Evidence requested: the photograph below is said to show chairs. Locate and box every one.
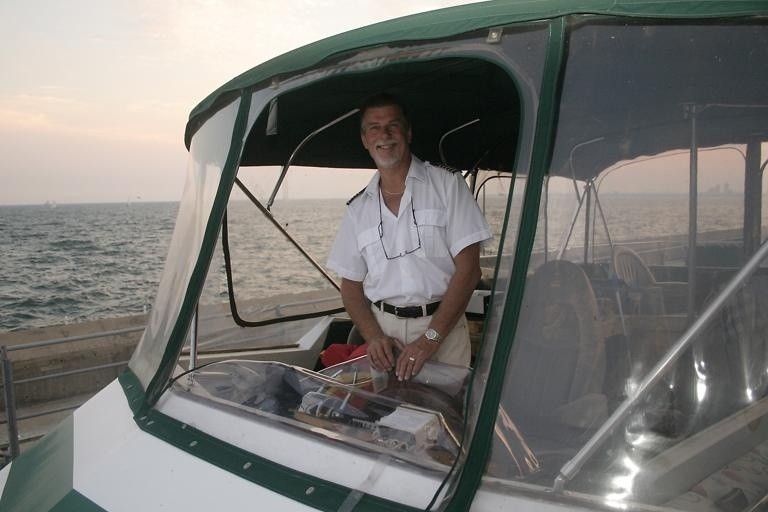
[492,258,608,480]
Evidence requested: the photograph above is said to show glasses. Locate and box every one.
[377,221,422,260]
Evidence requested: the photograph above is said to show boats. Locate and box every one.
[0,1,767,510]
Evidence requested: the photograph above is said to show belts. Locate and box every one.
[372,299,442,318]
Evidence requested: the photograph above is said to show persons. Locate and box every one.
[325,89,494,399]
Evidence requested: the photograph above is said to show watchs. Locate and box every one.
[424,327,443,344]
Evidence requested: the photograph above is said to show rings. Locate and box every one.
[409,357,415,362]
